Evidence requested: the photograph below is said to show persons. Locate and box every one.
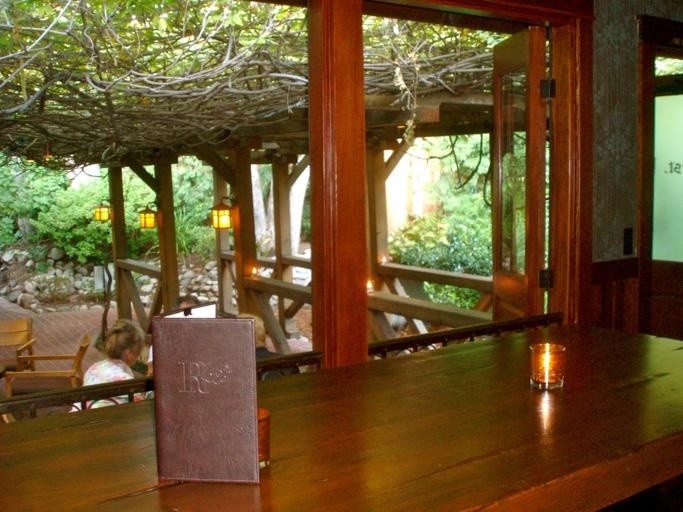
[66,318,145,416]
[236,313,301,380]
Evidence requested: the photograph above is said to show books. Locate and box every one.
[150,302,260,483]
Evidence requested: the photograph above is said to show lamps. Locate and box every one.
[90,196,160,229]
[208,192,238,231]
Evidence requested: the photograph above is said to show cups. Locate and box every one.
[530,342,566,390]
[257,407,271,469]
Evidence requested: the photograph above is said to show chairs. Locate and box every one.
[0,316,91,398]
